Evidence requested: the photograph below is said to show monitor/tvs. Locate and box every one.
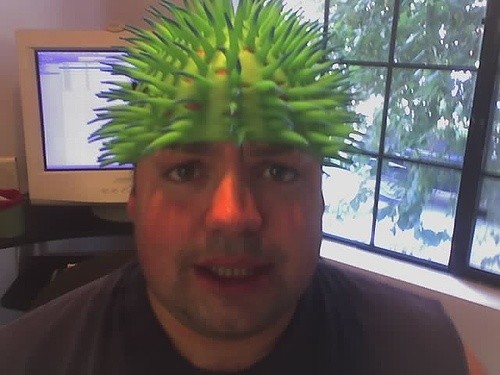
[15,28,153,223]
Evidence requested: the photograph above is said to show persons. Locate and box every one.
[0,0,469,375]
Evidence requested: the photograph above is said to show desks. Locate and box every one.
[0,205,139,314]
